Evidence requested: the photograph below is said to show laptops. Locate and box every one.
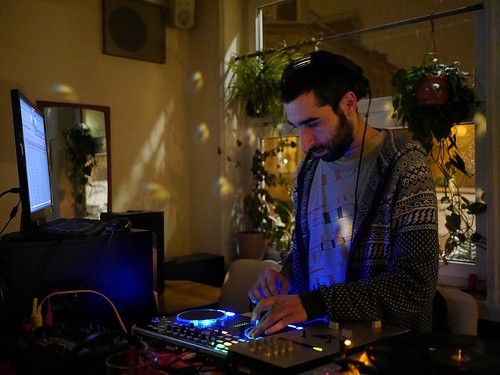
[10,88,107,237]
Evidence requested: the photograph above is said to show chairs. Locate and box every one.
[220,258,283,313]
[436,288,478,338]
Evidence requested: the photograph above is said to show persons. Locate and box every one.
[248,50,438,375]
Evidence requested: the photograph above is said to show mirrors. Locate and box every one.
[36,100,112,224]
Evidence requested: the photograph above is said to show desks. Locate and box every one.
[160,280,220,317]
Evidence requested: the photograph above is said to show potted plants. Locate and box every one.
[224,41,305,153]
[60,122,104,218]
[218,133,298,262]
[391,52,487,264]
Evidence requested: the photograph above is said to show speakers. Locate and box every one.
[169,0,195,29]
[100,210,166,297]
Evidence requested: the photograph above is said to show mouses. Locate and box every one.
[106,217,132,233]
